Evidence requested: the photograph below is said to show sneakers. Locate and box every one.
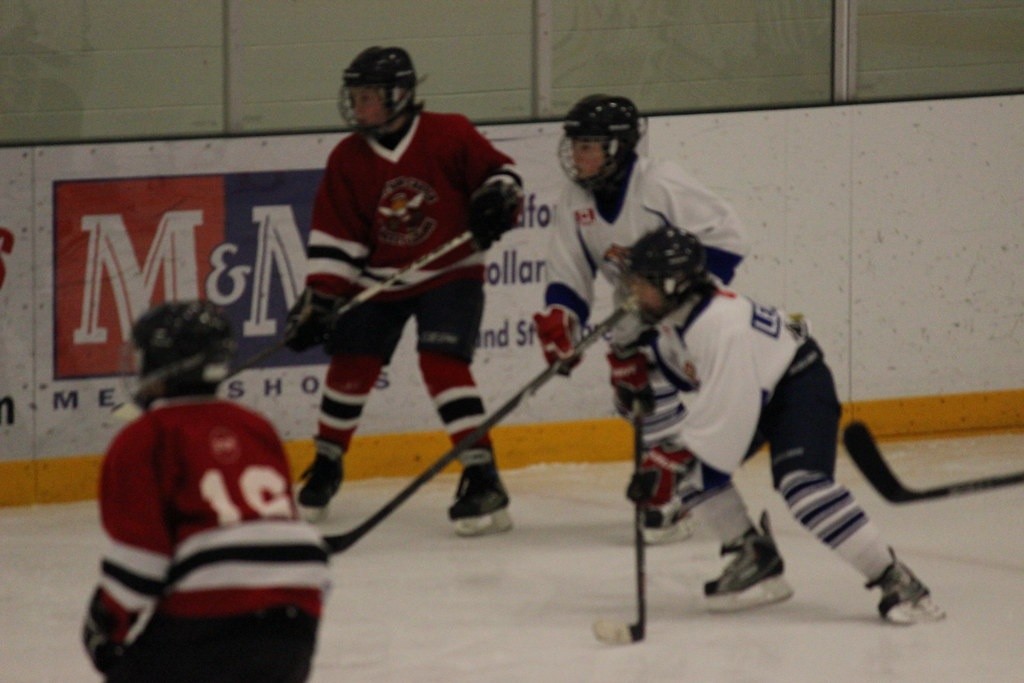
[866,545,944,623]
[635,494,692,543]
[294,435,345,521]
[447,447,510,535]
[702,510,794,613]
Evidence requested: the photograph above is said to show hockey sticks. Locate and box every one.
[843,421,1024,502]
[220,227,474,388]
[593,398,647,643]
[319,308,625,555]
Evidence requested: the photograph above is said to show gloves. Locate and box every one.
[534,305,582,374]
[282,286,344,349]
[628,440,696,504]
[467,176,523,251]
[608,349,655,413]
[83,616,121,671]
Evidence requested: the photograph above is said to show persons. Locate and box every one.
[282,45,526,536]
[84,299,333,683]
[533,94,750,543]
[626,224,947,627]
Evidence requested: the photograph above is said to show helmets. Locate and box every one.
[628,224,701,318]
[556,94,639,184]
[336,45,416,132]
[123,298,241,406]
